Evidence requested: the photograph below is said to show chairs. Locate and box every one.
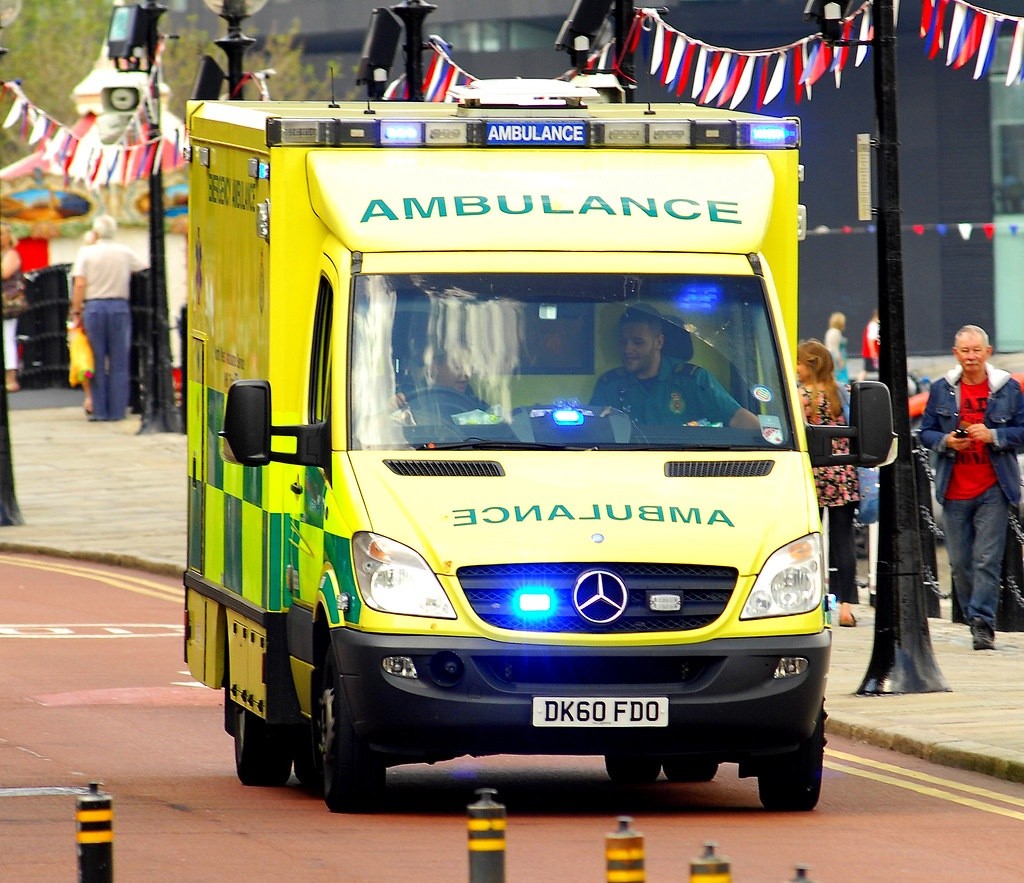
[660,313,692,359]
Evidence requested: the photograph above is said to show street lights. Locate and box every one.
[204,1,274,102]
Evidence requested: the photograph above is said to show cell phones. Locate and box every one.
[956,429,969,438]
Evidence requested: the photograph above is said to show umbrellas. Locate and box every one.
[0,112,187,216]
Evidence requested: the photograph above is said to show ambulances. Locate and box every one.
[179,74,899,809]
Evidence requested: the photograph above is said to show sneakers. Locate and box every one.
[969,616,997,650]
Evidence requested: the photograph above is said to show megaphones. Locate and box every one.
[102,72,149,114]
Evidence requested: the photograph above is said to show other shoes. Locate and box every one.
[838,612,859,627]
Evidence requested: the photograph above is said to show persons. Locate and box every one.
[0,223,23,392]
[68,215,152,422]
[366,336,519,441]
[586,303,760,429]
[858,309,879,382]
[825,313,850,383]
[797,340,861,627]
[918,324,1024,649]
